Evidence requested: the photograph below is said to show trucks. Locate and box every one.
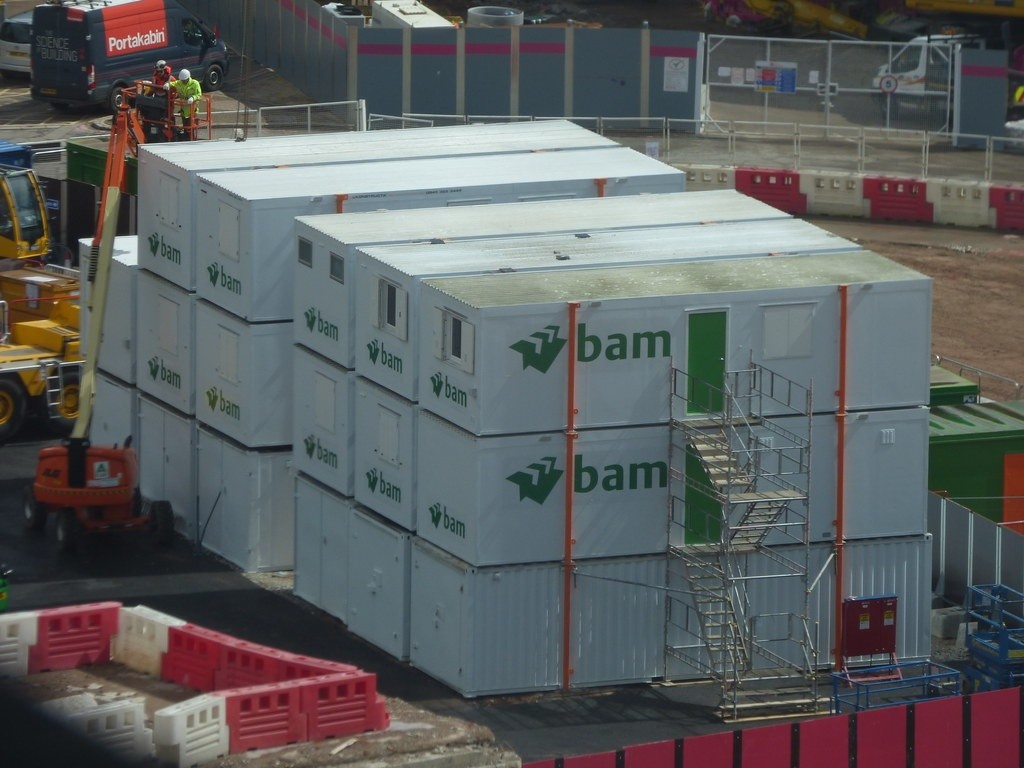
[876,35,988,105]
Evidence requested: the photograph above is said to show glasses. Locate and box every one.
[154,65,165,69]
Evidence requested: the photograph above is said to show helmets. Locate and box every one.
[156,60,166,71]
[178,69,190,81]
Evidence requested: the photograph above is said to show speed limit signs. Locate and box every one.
[881,75,897,93]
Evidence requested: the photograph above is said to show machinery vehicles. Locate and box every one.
[0,161,88,452]
[19,76,201,564]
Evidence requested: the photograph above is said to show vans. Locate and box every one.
[0,10,34,75]
[30,0,232,115]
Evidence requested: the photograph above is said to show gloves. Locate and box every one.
[163,83,170,91]
[187,97,193,105]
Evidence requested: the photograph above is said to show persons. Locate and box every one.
[163,69,202,134]
[146,59,176,125]
